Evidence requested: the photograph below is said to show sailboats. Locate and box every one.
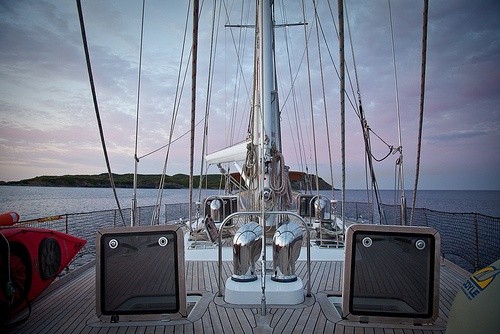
[0,0,499,334]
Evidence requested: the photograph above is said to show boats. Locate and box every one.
[0,226,88,322]
